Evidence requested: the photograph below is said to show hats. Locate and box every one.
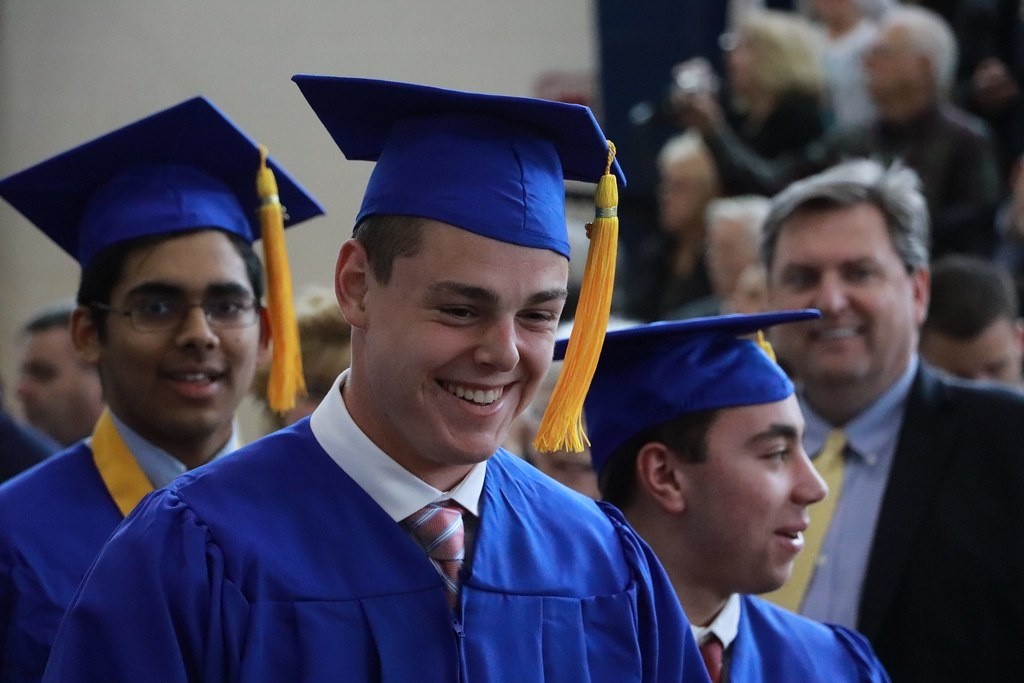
[1,95,327,416]
[554,308,822,475]
[291,74,628,453]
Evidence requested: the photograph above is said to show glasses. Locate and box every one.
[94,279,263,334]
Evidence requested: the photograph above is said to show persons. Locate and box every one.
[41,76,712,683]
[18,301,106,448]
[506,306,891,683]
[0,94,326,683]
[534,0,1024,682]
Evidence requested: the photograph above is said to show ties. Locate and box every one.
[698,636,723,683]
[758,428,846,614]
[404,503,471,615]
